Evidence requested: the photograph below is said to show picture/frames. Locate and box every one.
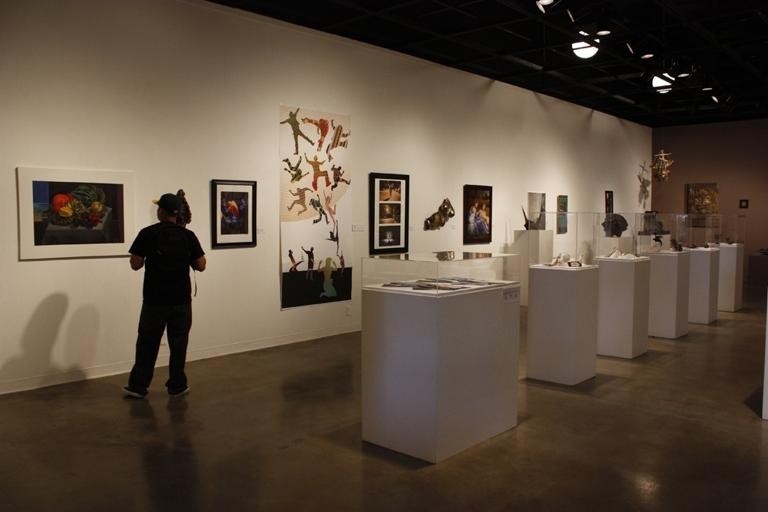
[16,164,137,261]
[367,172,410,255]
[211,178,257,250]
[462,183,494,246]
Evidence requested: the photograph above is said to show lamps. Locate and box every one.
[535,0,734,105]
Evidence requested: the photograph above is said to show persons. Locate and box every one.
[121,189,206,398]
[468,199,490,237]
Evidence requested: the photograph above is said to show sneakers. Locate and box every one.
[168,385,191,398]
[122,386,145,399]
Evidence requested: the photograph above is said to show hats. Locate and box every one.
[153,193,179,206]
